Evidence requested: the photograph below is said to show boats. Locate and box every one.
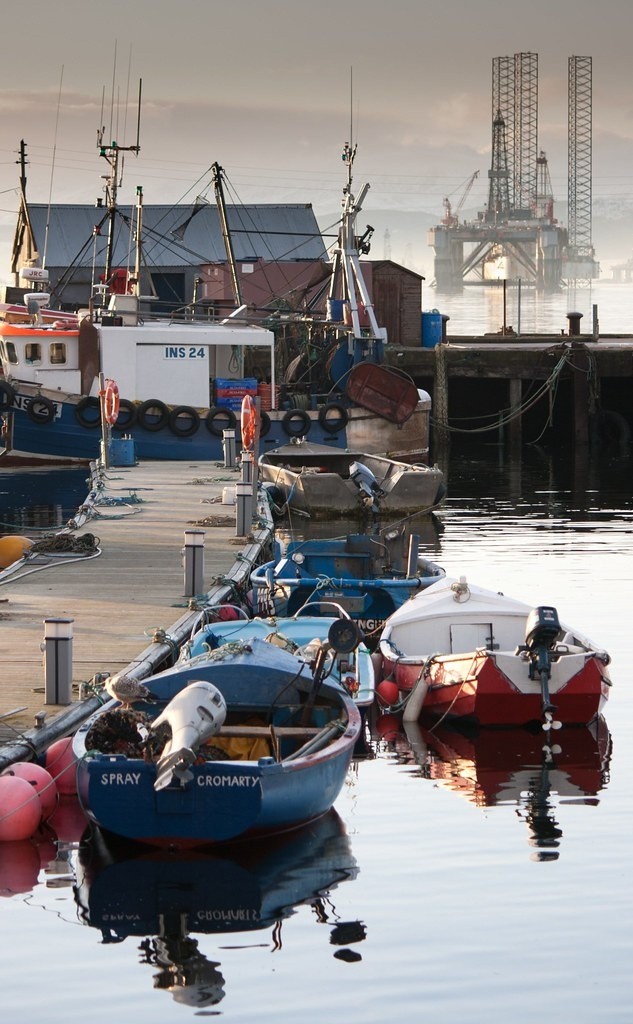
[1,34,433,467]
[369,574,615,732]
[70,633,364,857]
[175,602,375,715]
[245,519,448,654]
[257,433,448,522]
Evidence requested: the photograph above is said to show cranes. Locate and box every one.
[441,169,482,228]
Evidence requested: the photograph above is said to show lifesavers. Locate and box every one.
[138,399,170,432]
[76,396,102,428]
[113,399,138,430]
[0,380,15,410]
[206,407,237,436]
[283,408,312,438]
[27,396,54,424]
[259,409,271,437]
[169,406,200,437]
[267,486,283,518]
[53,319,88,328]
[319,402,349,434]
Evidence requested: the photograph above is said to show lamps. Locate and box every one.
[184,529,206,546]
[44,618,74,639]
[241,450,254,461]
[236,480,252,497]
[223,429,235,439]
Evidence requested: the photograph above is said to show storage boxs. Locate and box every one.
[326,299,375,326]
[213,378,321,414]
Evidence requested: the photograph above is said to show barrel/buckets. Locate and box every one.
[107,438,135,467]
[327,299,375,327]
[422,313,442,347]
